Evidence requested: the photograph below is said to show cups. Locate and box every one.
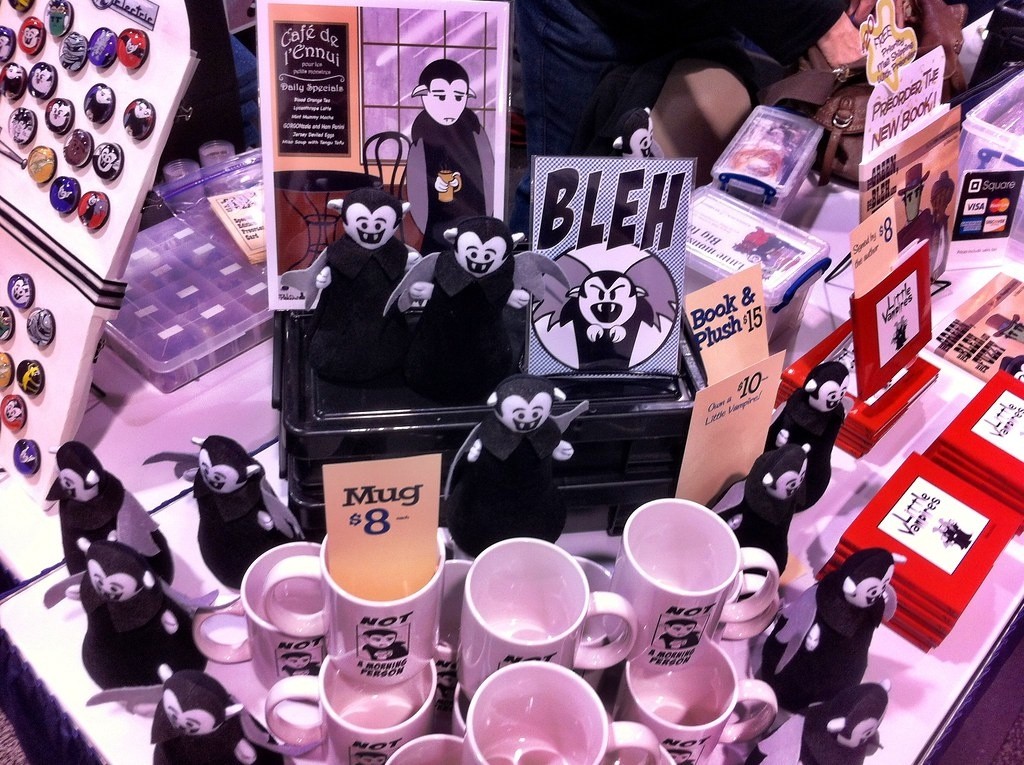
[274,169,383,276]
[194,498,780,765]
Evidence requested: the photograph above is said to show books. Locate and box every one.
[858,103,1024,282]
[923,272,1024,392]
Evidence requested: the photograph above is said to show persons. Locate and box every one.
[509,0,914,253]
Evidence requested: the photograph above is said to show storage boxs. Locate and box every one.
[682,184,832,345]
[100,215,275,393]
[968,0,1024,88]
[957,69,1024,285]
[710,104,824,219]
